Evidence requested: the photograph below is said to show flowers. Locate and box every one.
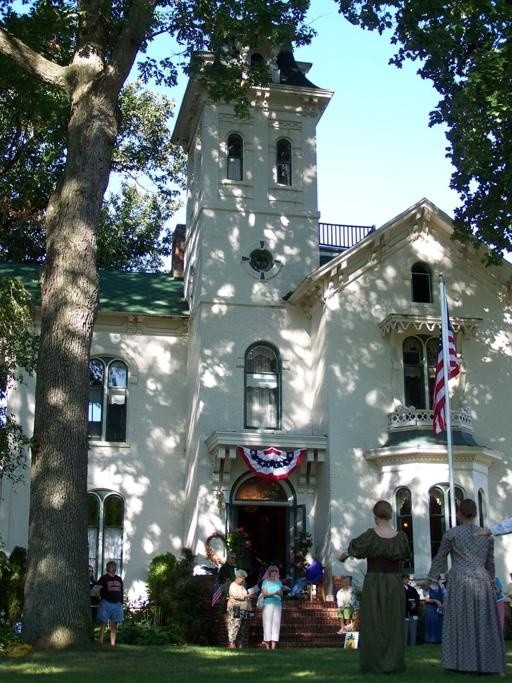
[287,531,313,578]
[226,526,252,570]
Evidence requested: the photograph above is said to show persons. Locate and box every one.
[94,561,124,647]
[422,496,508,675]
[491,575,506,634]
[284,552,322,600]
[87,564,100,625]
[472,514,512,539]
[224,567,256,648]
[335,575,357,634]
[421,574,445,643]
[260,562,288,649]
[400,573,421,647]
[216,552,237,618]
[333,499,416,674]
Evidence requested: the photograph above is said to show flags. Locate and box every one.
[432,292,461,436]
[211,585,222,607]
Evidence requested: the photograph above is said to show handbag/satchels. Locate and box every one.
[232,604,241,619]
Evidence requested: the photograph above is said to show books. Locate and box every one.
[247,583,260,594]
[279,584,291,590]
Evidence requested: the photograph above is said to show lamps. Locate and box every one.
[403,518,409,527]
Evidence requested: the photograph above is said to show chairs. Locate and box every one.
[308,567,327,602]
